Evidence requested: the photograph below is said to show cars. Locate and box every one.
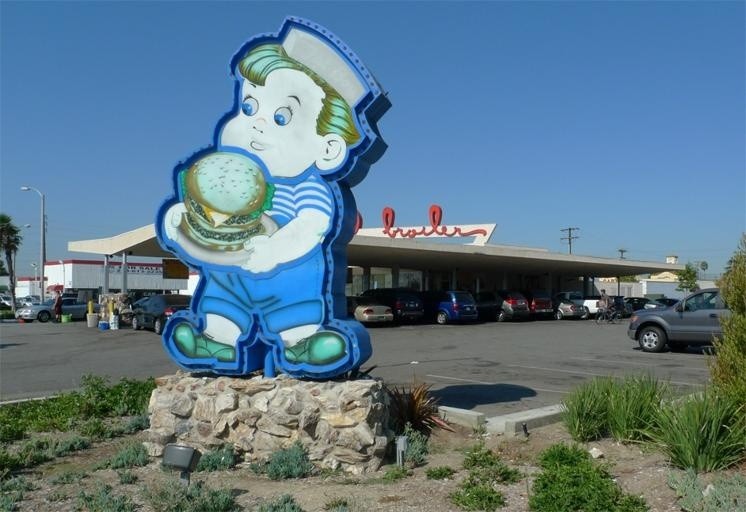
[0,292,155,323]
[624,287,731,354]
[128,293,194,335]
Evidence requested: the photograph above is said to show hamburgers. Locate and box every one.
[181,150,274,251]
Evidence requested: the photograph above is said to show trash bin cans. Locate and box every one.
[87,314,99,327]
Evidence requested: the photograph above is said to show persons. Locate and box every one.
[153,16,393,379]
[52,291,63,322]
[599,288,615,322]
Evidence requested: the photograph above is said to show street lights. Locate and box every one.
[56,259,65,293]
[29,261,39,295]
[12,220,31,285]
[19,185,46,303]
